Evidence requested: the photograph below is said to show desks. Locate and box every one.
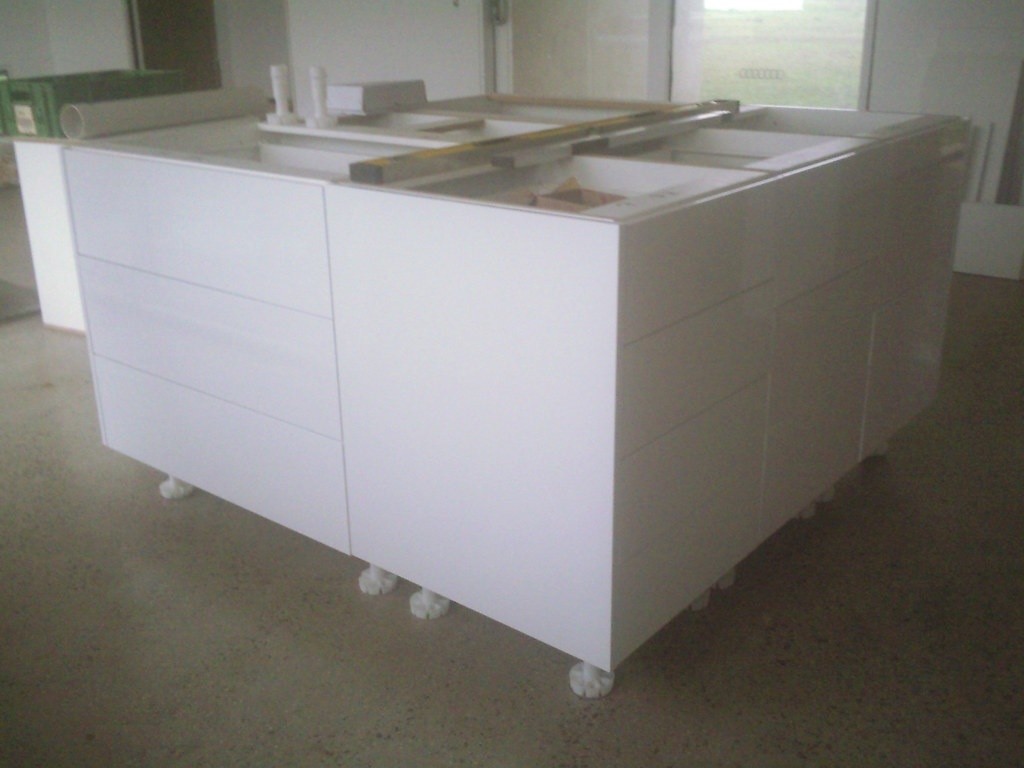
[60,110,979,675]
